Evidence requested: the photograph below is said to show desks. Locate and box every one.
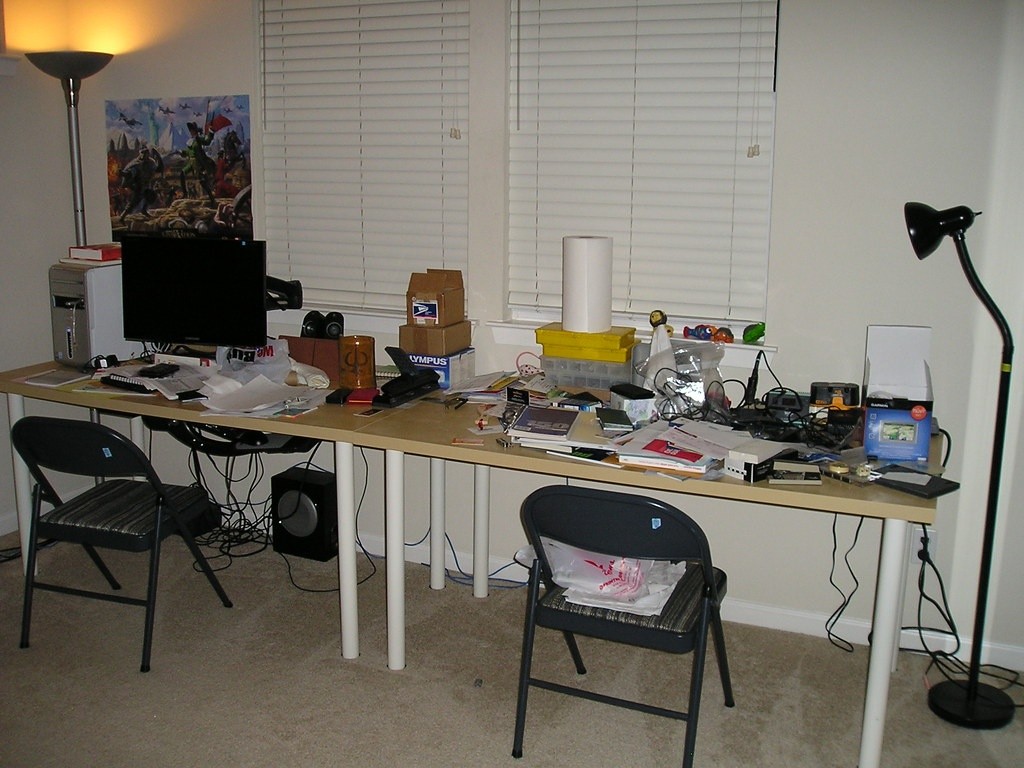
[1,346,949,768]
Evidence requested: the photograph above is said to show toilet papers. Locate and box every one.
[562,234,614,334]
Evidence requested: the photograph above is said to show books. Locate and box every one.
[69,243,122,262]
[92,360,208,403]
[444,371,744,483]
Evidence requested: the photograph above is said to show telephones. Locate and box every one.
[372,368,442,408]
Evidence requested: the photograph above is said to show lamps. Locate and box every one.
[905,200,1017,730]
[23,50,114,266]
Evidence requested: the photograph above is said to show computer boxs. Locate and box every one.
[48,263,155,366]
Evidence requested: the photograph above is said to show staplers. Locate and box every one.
[767,459,823,486]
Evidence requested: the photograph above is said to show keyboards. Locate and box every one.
[187,422,246,443]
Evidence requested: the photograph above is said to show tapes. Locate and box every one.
[829,460,850,473]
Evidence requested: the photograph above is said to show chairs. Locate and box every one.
[512,484,735,767]
[8,416,234,672]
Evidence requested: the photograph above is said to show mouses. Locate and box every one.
[247,432,268,446]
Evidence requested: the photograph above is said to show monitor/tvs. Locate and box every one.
[118,231,302,349]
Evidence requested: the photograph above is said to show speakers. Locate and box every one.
[270,467,339,561]
[299,310,344,339]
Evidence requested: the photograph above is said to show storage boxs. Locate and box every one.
[398,268,476,388]
[862,325,934,461]
[536,322,642,392]
